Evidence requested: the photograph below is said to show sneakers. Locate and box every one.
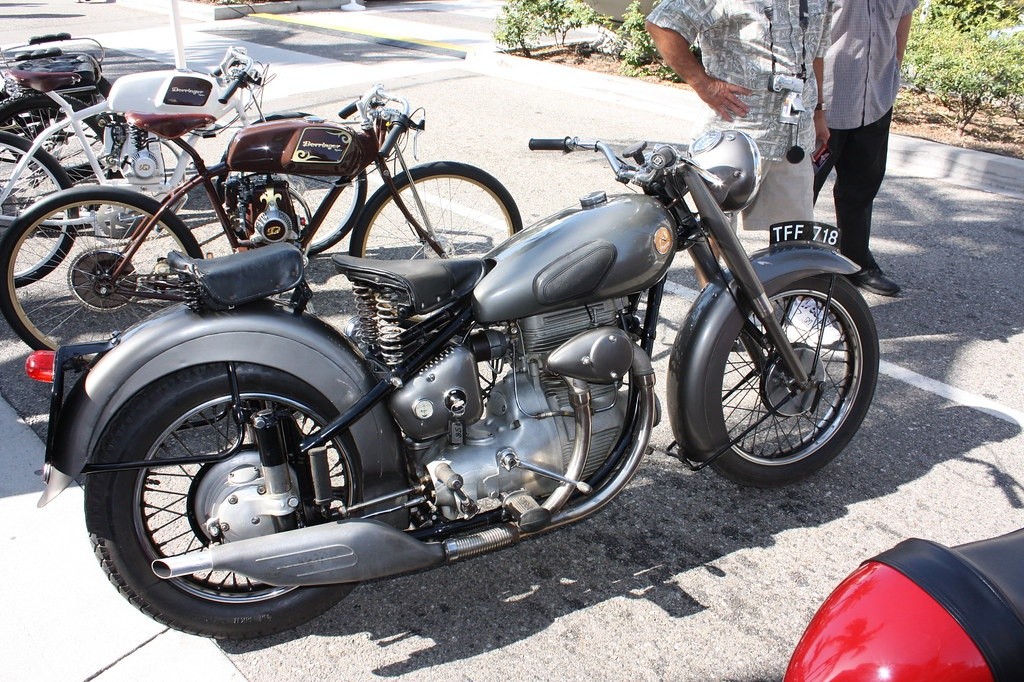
[789,298,840,348]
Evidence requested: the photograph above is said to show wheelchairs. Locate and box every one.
[0,32,128,230]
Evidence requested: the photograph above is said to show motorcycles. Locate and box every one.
[25,129,881,639]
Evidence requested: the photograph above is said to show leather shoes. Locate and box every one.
[849,263,901,296]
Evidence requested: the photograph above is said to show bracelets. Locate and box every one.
[815,103,826,111]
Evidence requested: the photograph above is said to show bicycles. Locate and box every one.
[0,46,368,288]
[1,82,522,366]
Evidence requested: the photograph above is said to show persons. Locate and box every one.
[641,0,844,353]
[809,0,923,294]
